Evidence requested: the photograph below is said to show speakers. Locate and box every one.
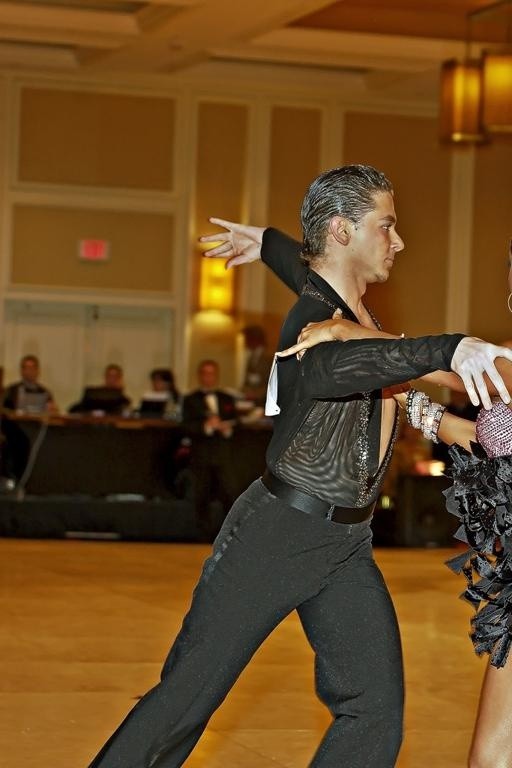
[395,475,461,545]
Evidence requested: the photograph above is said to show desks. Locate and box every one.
[1,409,462,554]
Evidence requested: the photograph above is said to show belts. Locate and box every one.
[259,466,380,528]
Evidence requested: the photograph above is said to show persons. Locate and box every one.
[431,392,488,460]
[86,165,512,768]
[1,325,277,426]
[270,242,512,768]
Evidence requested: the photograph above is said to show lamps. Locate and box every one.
[441,0,486,146]
[476,0,512,134]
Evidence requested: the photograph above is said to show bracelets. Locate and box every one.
[406,383,448,446]
